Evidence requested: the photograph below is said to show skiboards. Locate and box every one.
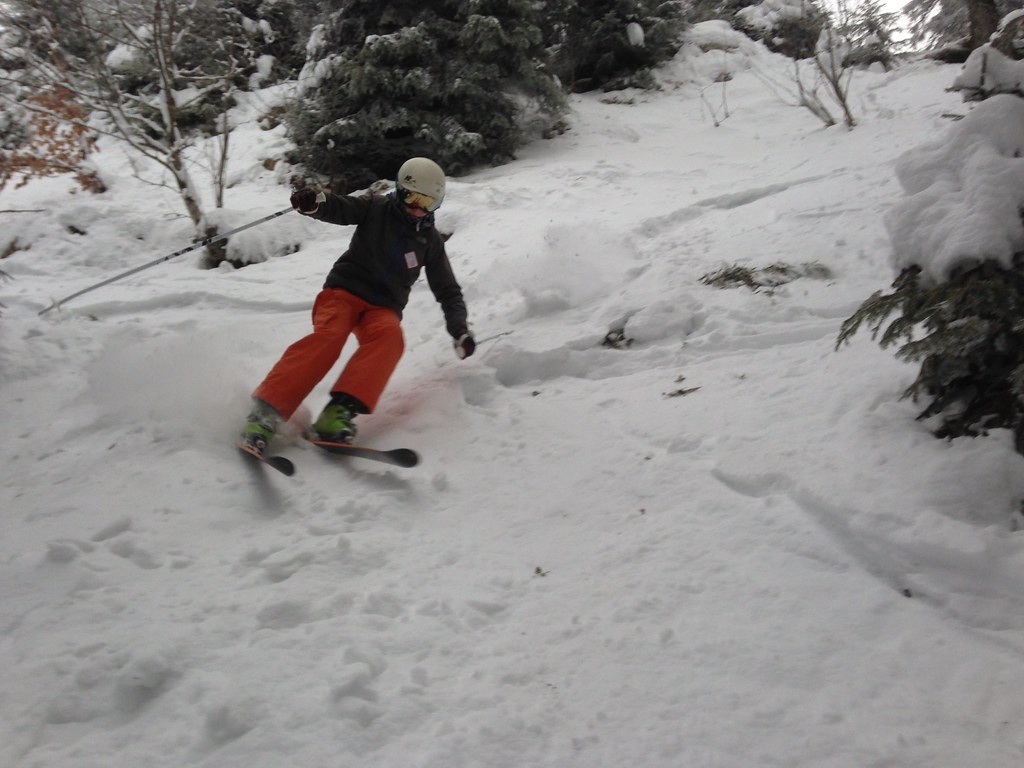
[234,435,422,477]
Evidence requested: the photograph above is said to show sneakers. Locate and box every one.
[311,397,363,441]
[241,402,278,444]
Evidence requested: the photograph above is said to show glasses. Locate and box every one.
[402,191,441,213]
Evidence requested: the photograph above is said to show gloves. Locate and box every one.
[291,188,318,213]
[448,320,476,361]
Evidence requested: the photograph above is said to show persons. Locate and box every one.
[239,157,476,456]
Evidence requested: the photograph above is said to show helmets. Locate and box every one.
[398,157,446,203]
